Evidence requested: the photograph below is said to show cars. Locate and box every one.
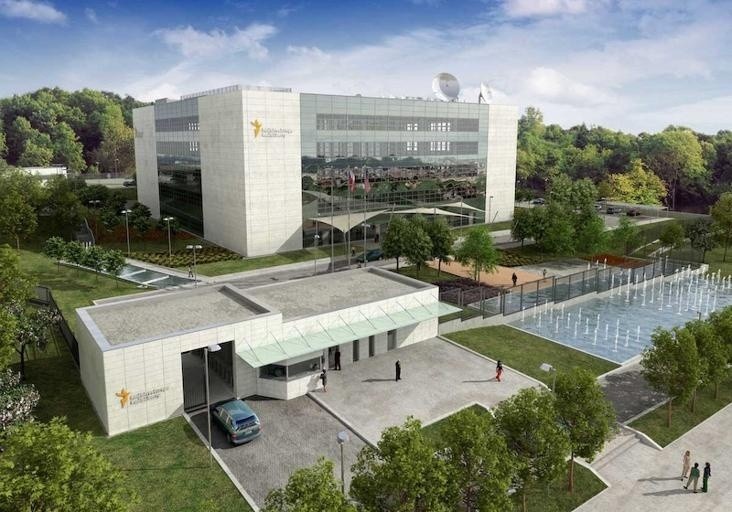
[357,249,385,263]
[303,165,474,189]
[594,204,602,210]
[213,400,263,444]
[534,198,546,204]
[607,207,623,213]
[627,209,640,216]
[123,181,136,187]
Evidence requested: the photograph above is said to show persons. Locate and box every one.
[680,450,691,481]
[543,268,547,277]
[320,368,329,392]
[333,348,341,370]
[683,462,700,493]
[699,462,711,492]
[511,272,518,286]
[395,359,404,382]
[494,359,505,382]
[312,363,319,371]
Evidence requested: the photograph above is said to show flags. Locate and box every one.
[364,167,371,194]
[348,166,357,194]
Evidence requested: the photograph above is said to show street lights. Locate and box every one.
[362,223,371,267]
[121,209,133,256]
[337,432,347,504]
[90,200,100,245]
[314,235,320,275]
[163,217,174,263]
[186,245,202,286]
[204,345,222,468]
[540,363,556,393]
